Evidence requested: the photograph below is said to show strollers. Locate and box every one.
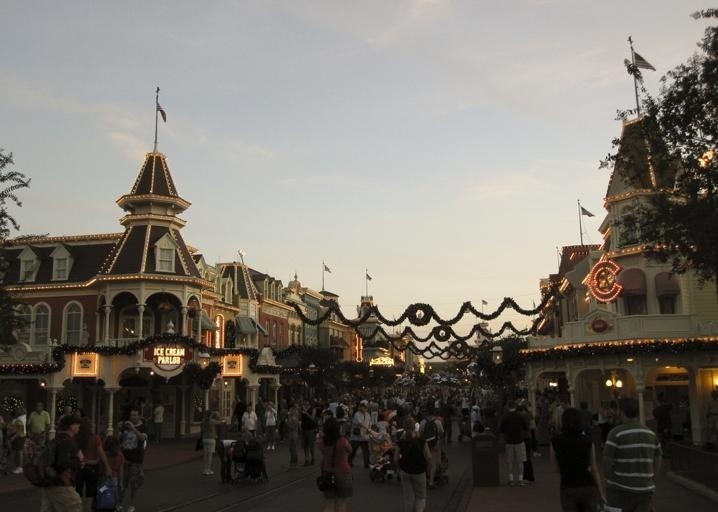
[366,430,399,484]
[229,436,268,487]
[455,413,471,442]
[427,449,450,488]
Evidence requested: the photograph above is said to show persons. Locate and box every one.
[8,400,164,512]
[548,396,672,512]
[200,386,537,510]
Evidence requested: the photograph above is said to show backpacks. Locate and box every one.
[24,438,74,488]
[422,418,439,446]
[93,475,118,512]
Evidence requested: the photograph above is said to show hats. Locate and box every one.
[59,414,83,429]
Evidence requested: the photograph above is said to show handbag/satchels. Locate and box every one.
[315,474,337,492]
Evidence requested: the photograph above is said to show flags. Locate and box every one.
[483,300,488,305]
[324,265,331,273]
[633,51,657,72]
[581,206,595,218]
[367,274,372,280]
[157,96,166,123]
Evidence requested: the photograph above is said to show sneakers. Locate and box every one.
[506,479,536,487]
[201,468,214,476]
[12,466,24,474]
[127,505,137,512]
